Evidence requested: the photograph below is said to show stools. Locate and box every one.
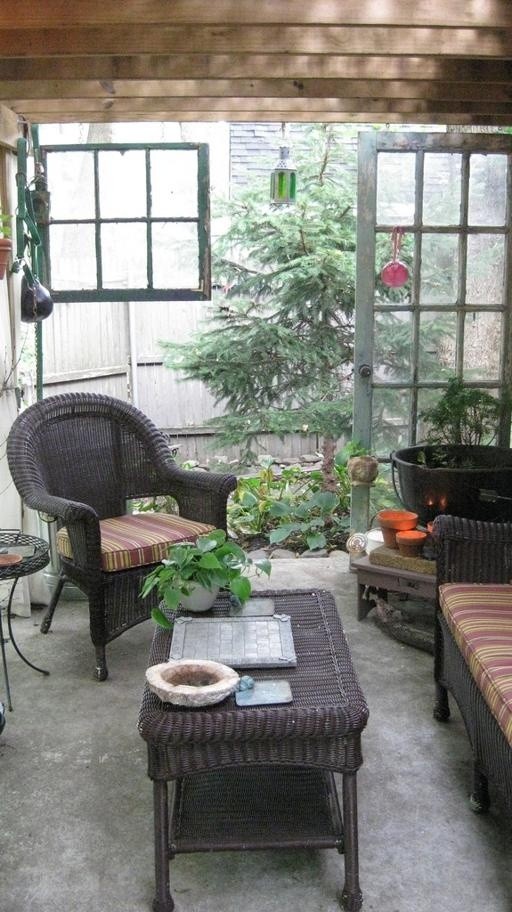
[351,555,436,622]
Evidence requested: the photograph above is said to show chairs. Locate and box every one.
[7,390,237,682]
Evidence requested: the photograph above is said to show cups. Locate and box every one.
[393,529,427,553]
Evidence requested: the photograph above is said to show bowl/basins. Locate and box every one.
[144,659,242,709]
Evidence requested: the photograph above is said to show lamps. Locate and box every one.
[268,146,297,206]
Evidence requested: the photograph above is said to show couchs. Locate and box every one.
[429,515,512,814]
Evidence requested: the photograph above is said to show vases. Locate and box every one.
[395,531,427,557]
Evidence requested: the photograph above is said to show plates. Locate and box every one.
[0,553,23,567]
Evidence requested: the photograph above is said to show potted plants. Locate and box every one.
[390,375,512,531]
[136,528,272,628]
[0,207,14,281]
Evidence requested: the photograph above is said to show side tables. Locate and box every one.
[0,531,55,715]
